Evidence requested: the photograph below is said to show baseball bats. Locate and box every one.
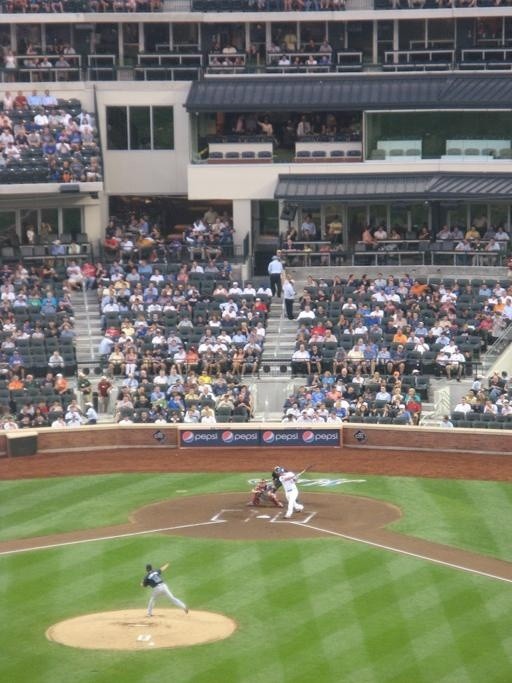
[296,465,312,476]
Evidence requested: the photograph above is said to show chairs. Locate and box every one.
[1,96,101,184]
[207,121,361,161]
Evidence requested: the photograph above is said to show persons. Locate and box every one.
[248,0,345,11]
[140,562,188,617]
[282,215,512,427]
[233,113,362,144]
[2,41,76,82]
[205,23,333,66]
[274,466,304,519]
[1,206,272,431]
[268,250,285,297]
[0,87,102,182]
[3,1,161,13]
[245,479,283,507]
[392,1,502,8]
[271,464,298,503]
